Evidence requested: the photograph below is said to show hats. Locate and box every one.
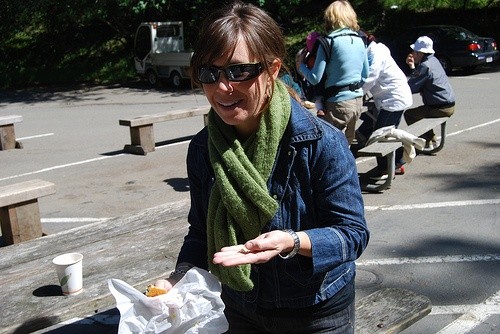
[410,36,435,53]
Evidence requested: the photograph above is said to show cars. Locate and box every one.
[382,23,500,75]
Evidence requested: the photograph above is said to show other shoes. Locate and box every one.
[368,168,388,180]
[395,164,404,174]
[424,140,433,151]
[317,110,325,115]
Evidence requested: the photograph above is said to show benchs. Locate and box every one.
[0,115,24,151]
[354,117,450,189]
[0,178,58,244]
[355,287,434,334]
[119,106,213,155]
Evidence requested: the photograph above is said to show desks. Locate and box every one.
[0,199,196,334]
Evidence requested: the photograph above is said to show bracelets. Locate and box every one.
[278,229,300,260]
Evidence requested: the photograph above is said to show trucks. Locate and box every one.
[131,19,195,90]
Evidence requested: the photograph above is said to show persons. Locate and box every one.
[280,0,455,175]
[153,4,370,334]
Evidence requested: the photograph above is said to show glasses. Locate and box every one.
[195,61,272,85]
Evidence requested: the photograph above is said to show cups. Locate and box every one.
[52,252,83,296]
